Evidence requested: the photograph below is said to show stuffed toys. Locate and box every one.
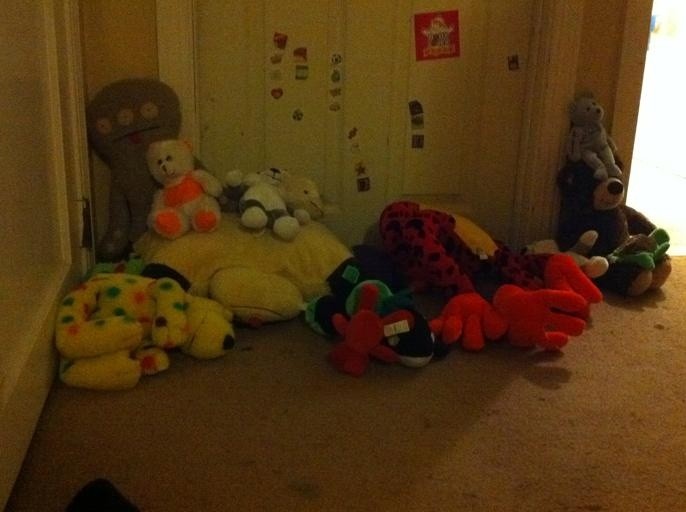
[51,77,671,391]
